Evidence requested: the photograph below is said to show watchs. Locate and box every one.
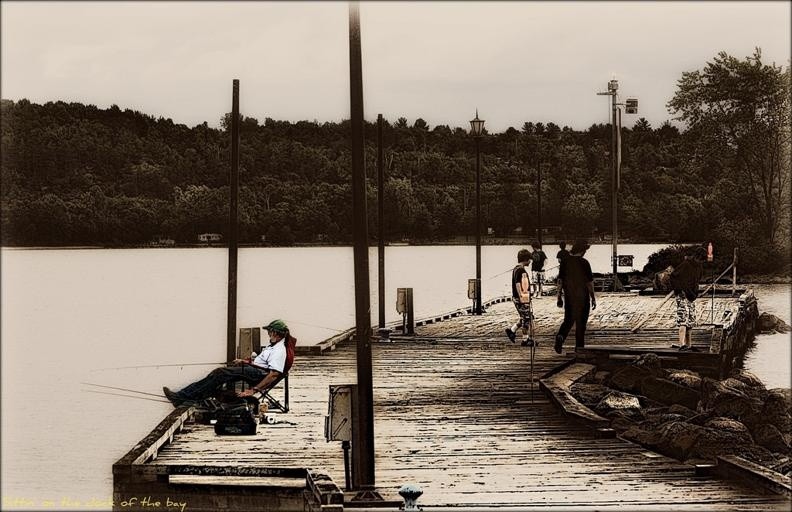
[253,385,259,392]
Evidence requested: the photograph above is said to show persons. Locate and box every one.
[668,246,707,352]
[556,242,568,264]
[555,240,596,353]
[505,249,538,347]
[529,241,548,298]
[162,318,290,408]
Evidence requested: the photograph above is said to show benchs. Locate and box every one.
[544,277,613,292]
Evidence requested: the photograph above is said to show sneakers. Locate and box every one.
[556,335,563,354]
[163,386,184,408]
[505,328,516,344]
[521,338,538,346]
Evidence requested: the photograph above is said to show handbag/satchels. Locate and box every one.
[214,406,257,435]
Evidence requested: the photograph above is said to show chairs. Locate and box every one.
[227,330,296,413]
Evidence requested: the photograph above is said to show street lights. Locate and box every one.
[470,107,486,317]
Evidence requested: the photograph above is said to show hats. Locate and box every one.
[570,243,590,254]
[262,319,288,331]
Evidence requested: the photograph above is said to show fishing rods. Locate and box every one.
[489,264,513,279]
[85,359,253,370]
[277,319,356,336]
[79,380,224,413]
